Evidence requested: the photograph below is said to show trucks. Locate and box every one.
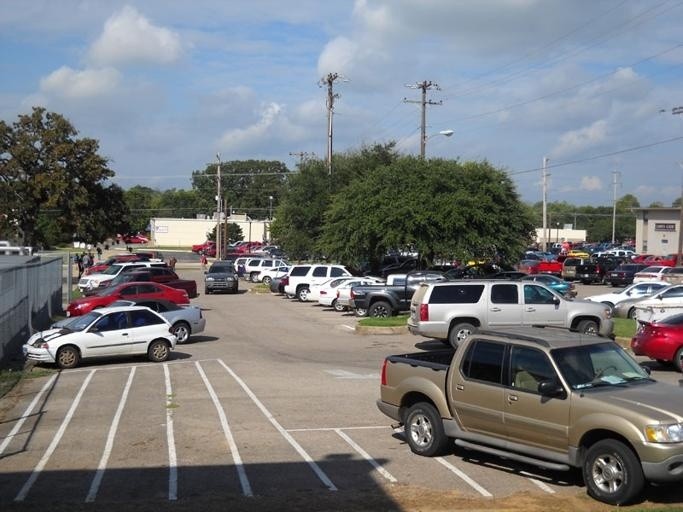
[379,332,683,503]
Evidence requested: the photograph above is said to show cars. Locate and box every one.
[0,229,209,366]
[584,265,683,374]
[443,241,682,299]
[192,237,451,316]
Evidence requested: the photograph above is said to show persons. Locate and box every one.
[72,248,95,280]
[598,306,616,339]
[166,255,177,272]
[198,251,209,272]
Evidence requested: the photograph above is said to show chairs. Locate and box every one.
[515,358,546,392]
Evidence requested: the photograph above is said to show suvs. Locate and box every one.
[410,281,615,348]
